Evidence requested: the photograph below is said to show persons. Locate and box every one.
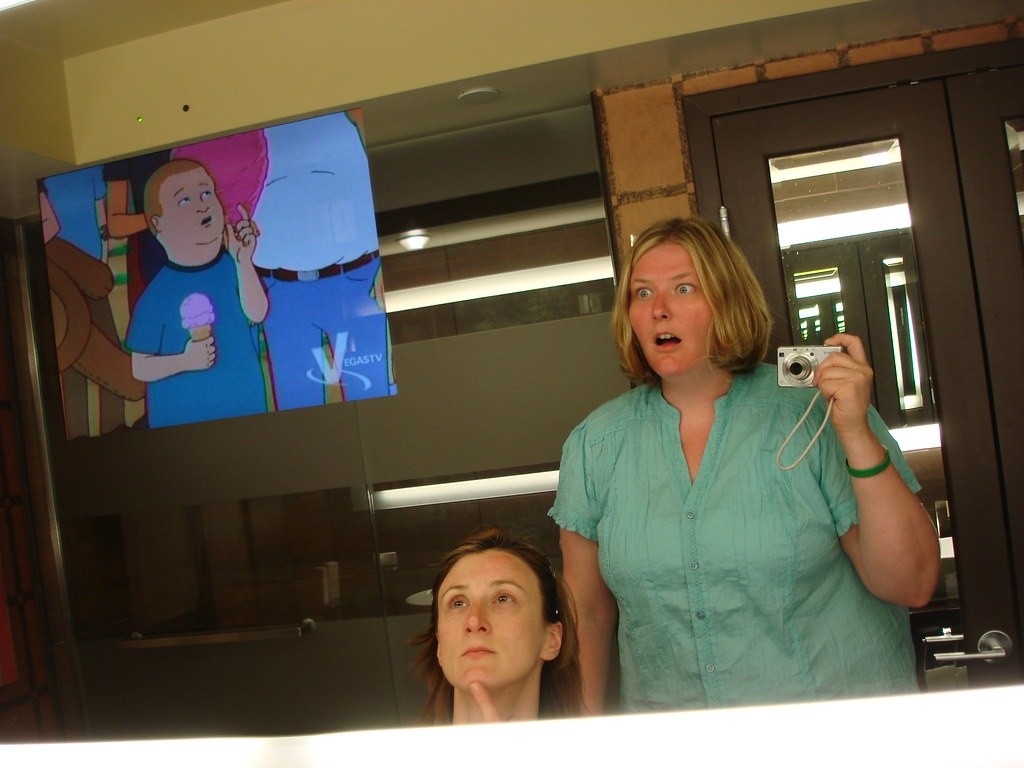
[418,527,585,726]
[548,217,939,718]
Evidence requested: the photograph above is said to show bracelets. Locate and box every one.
[846,445,890,477]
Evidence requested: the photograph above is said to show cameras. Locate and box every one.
[777,344,846,388]
